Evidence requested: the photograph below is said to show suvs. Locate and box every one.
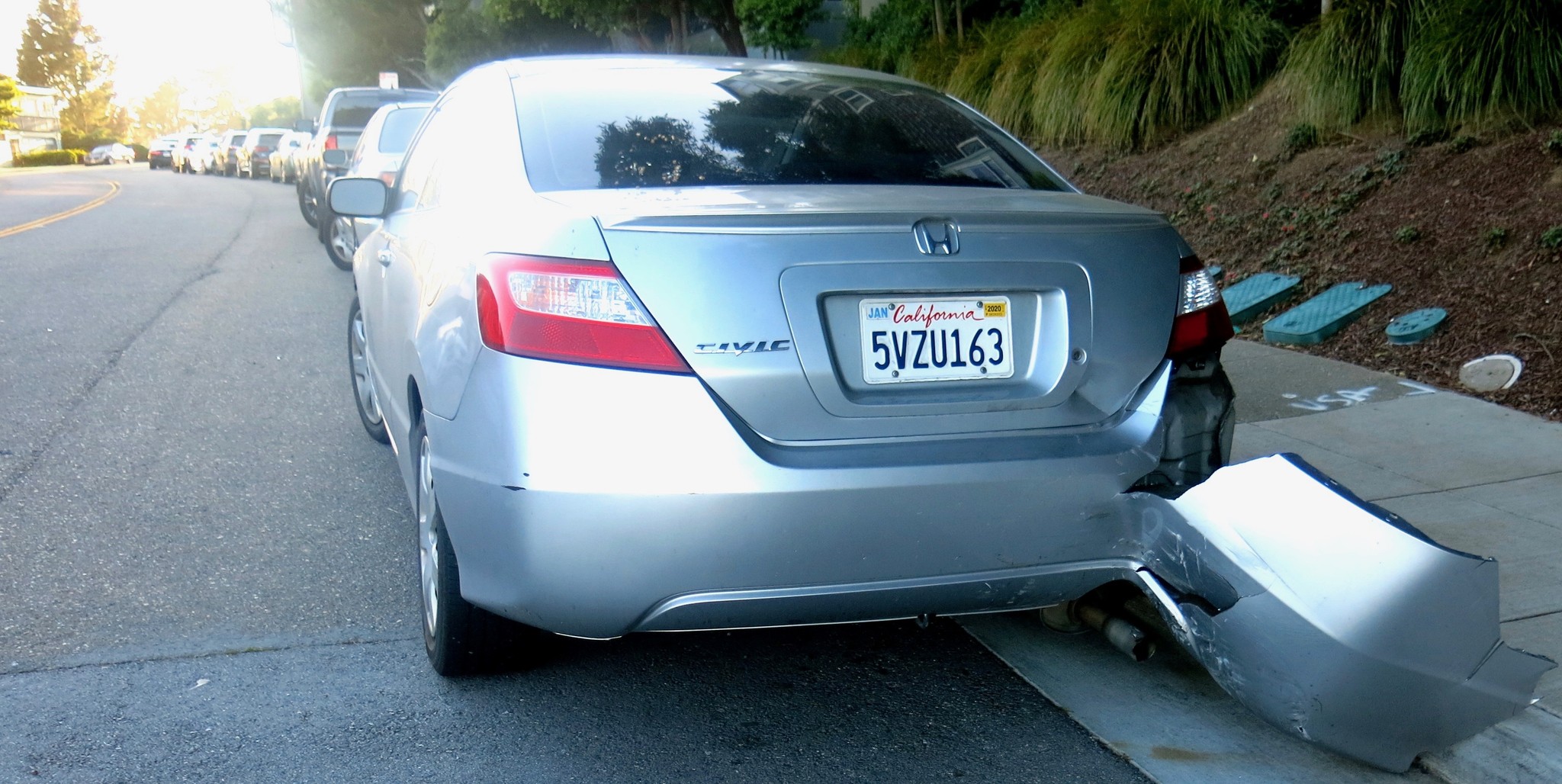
[293,83,445,245]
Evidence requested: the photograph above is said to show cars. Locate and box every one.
[317,96,441,272]
[144,120,314,185]
[321,48,1558,777]
[83,142,136,166]
